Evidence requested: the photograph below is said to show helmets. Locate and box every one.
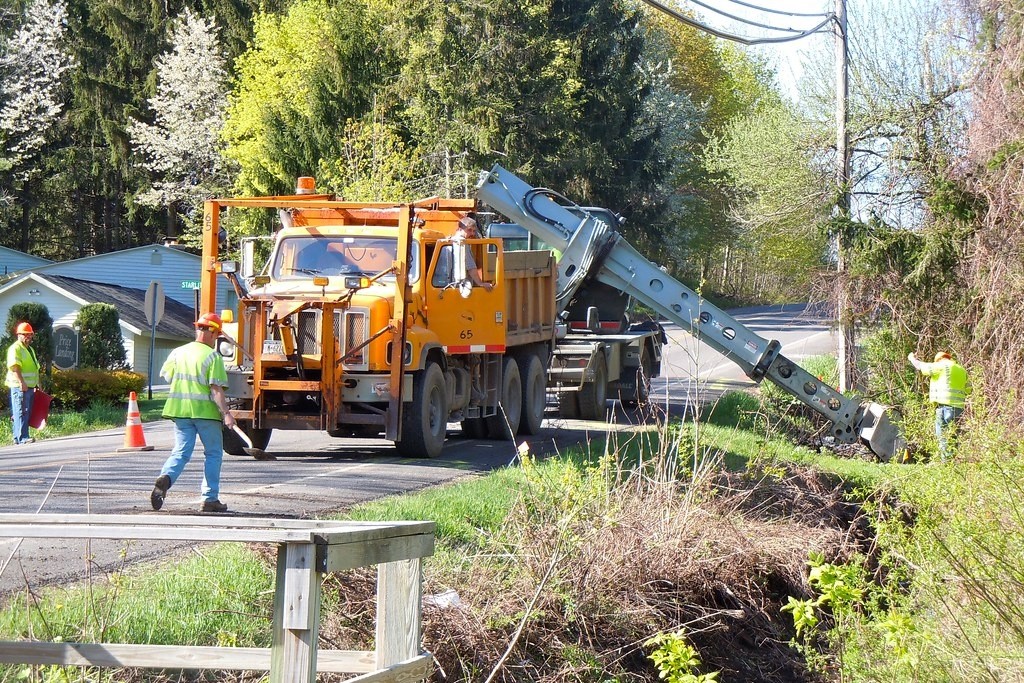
[193,312,224,336]
[934,351,953,362]
[16,323,34,334]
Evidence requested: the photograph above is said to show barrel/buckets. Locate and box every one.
[11,388,52,430]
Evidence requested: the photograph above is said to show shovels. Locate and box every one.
[233,425,277,461]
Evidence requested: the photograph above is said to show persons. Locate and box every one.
[432,217,495,293]
[908,352,973,463]
[5,323,42,444]
[150,312,237,512]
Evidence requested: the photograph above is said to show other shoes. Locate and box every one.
[13,437,36,445]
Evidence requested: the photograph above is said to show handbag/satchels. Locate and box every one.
[10,387,53,429]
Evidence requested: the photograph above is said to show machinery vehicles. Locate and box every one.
[487,207,909,462]
[200,177,557,459]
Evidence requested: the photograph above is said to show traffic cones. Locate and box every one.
[117,391,154,452]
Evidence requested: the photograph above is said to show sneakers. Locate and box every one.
[200,500,228,512]
[151,475,171,510]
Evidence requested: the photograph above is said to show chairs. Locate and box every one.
[324,252,344,270]
